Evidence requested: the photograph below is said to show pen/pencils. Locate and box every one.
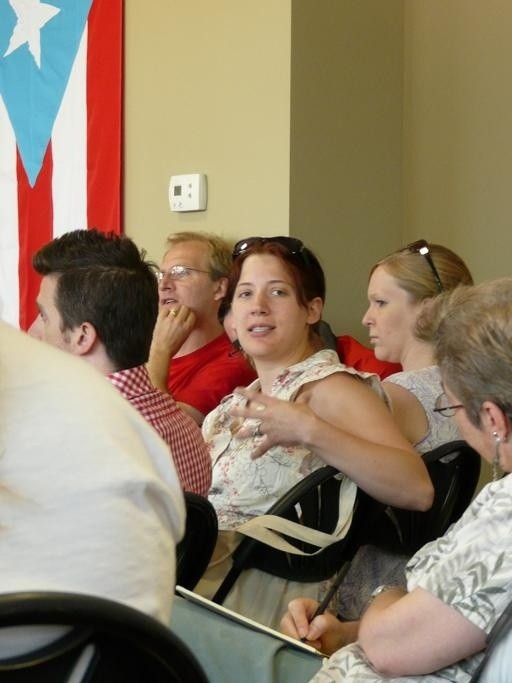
[300,560,351,643]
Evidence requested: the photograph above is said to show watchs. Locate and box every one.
[368,584,405,602]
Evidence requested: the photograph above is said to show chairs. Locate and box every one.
[0,590,209,682]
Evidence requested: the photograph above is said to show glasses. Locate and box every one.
[231,236,305,261]
[433,392,464,417]
[154,264,211,285]
[407,238,444,292]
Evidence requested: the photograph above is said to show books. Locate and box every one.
[175,582,331,661]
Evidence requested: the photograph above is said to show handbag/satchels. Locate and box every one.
[192,529,339,633]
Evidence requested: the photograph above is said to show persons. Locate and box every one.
[358,236,478,456]
[305,274,511,683]
[19,225,216,505]
[218,290,404,381]
[146,227,263,432]
[0,302,189,681]
[198,234,436,531]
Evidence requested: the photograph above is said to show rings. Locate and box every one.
[169,308,179,316]
[252,423,264,438]
